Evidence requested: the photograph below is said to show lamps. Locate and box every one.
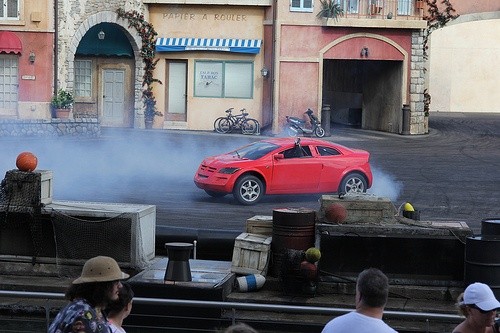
[261,66,271,79]
[98,24,105,39]
[29,50,36,63]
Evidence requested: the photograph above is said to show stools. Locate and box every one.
[164,242,194,282]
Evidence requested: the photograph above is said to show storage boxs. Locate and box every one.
[4,169,54,208]
[314,219,471,286]
[230,215,273,278]
[320,194,391,224]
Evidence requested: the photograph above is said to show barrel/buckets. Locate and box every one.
[272,208,317,276]
[463,218,500,303]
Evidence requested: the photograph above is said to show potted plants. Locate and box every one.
[317,0,345,23]
[50,89,75,118]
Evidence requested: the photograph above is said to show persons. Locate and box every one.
[449,284,500,333]
[48,255,132,333]
[218,323,258,333]
[321,268,399,333]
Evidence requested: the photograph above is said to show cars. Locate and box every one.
[193,135,373,206]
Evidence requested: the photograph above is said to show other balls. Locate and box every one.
[16,151,39,172]
[324,203,347,225]
[300,260,317,281]
[304,248,323,263]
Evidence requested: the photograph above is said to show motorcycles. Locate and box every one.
[282,108,325,137]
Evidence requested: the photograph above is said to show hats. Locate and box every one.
[72,255,130,284]
[458,282,500,311]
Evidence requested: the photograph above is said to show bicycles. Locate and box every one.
[214,107,260,135]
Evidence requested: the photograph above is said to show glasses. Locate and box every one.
[469,304,496,313]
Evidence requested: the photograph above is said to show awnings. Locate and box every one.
[155,36,262,55]
[0,31,23,56]
[74,23,133,58]
